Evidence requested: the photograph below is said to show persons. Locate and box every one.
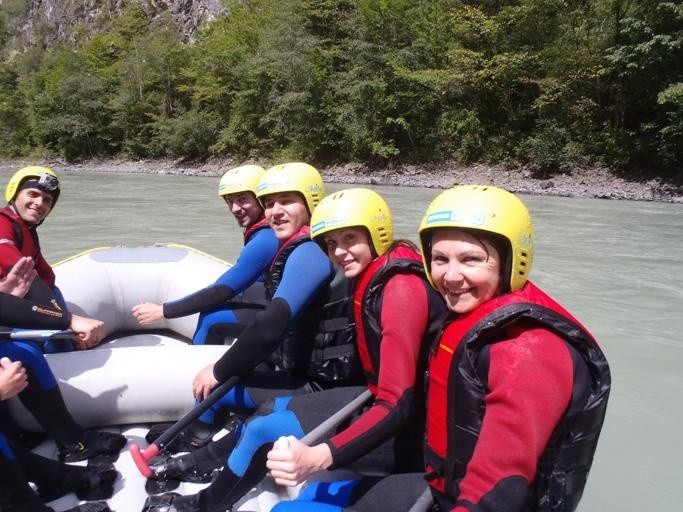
[144,162,327,444]
[130,163,282,347]
[268,184,611,512]
[0,166,93,353]
[0,255,127,511]
[144,189,445,512]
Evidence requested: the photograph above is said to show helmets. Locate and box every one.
[310,188,393,257]
[219,162,326,215]
[418,185,534,292]
[5,166,62,217]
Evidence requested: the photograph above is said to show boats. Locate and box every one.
[0,244,367,512]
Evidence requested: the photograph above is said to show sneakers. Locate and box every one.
[142,424,232,512]
[36,430,127,512]
